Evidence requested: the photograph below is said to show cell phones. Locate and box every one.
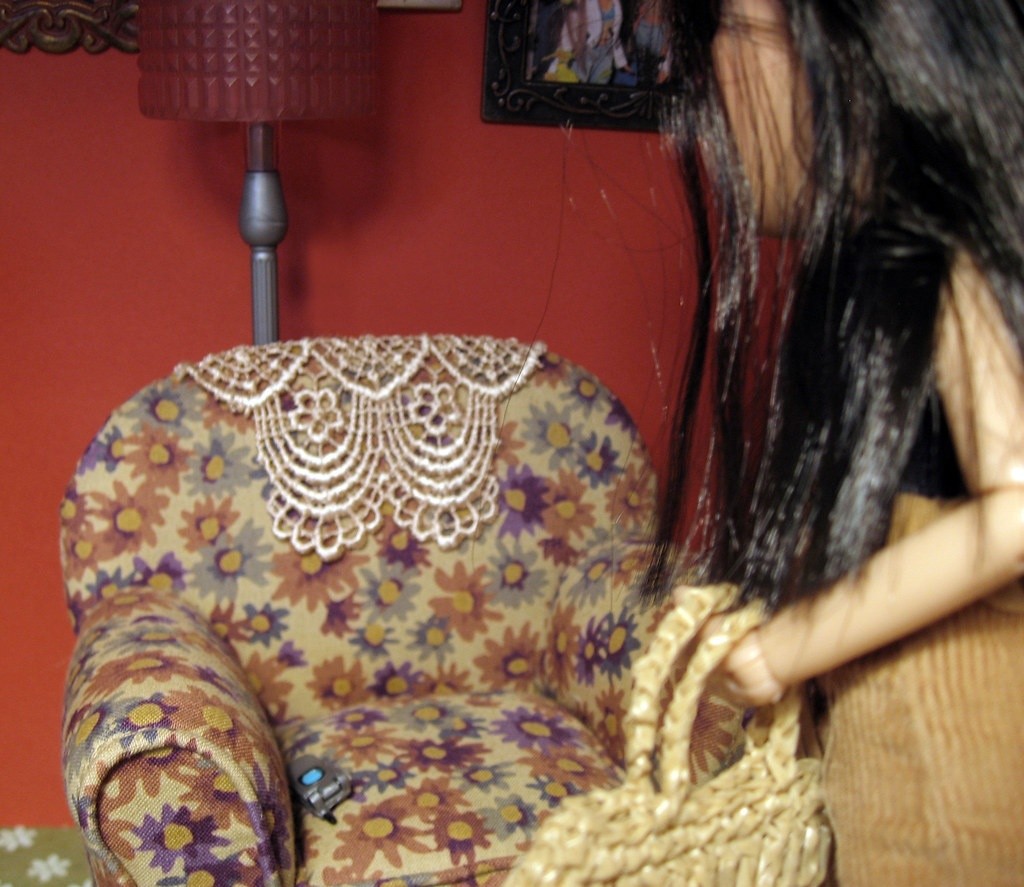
[286,754,355,826]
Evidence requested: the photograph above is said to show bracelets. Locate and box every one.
[723,623,785,708]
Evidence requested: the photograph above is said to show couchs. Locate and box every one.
[57,335,823,887]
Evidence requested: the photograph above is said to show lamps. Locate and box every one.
[137,0,380,343]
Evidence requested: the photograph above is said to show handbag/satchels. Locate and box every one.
[500,582,832,887]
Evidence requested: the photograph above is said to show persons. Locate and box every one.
[632,2,1021,883]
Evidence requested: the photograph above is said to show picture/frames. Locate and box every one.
[479,0,673,133]
